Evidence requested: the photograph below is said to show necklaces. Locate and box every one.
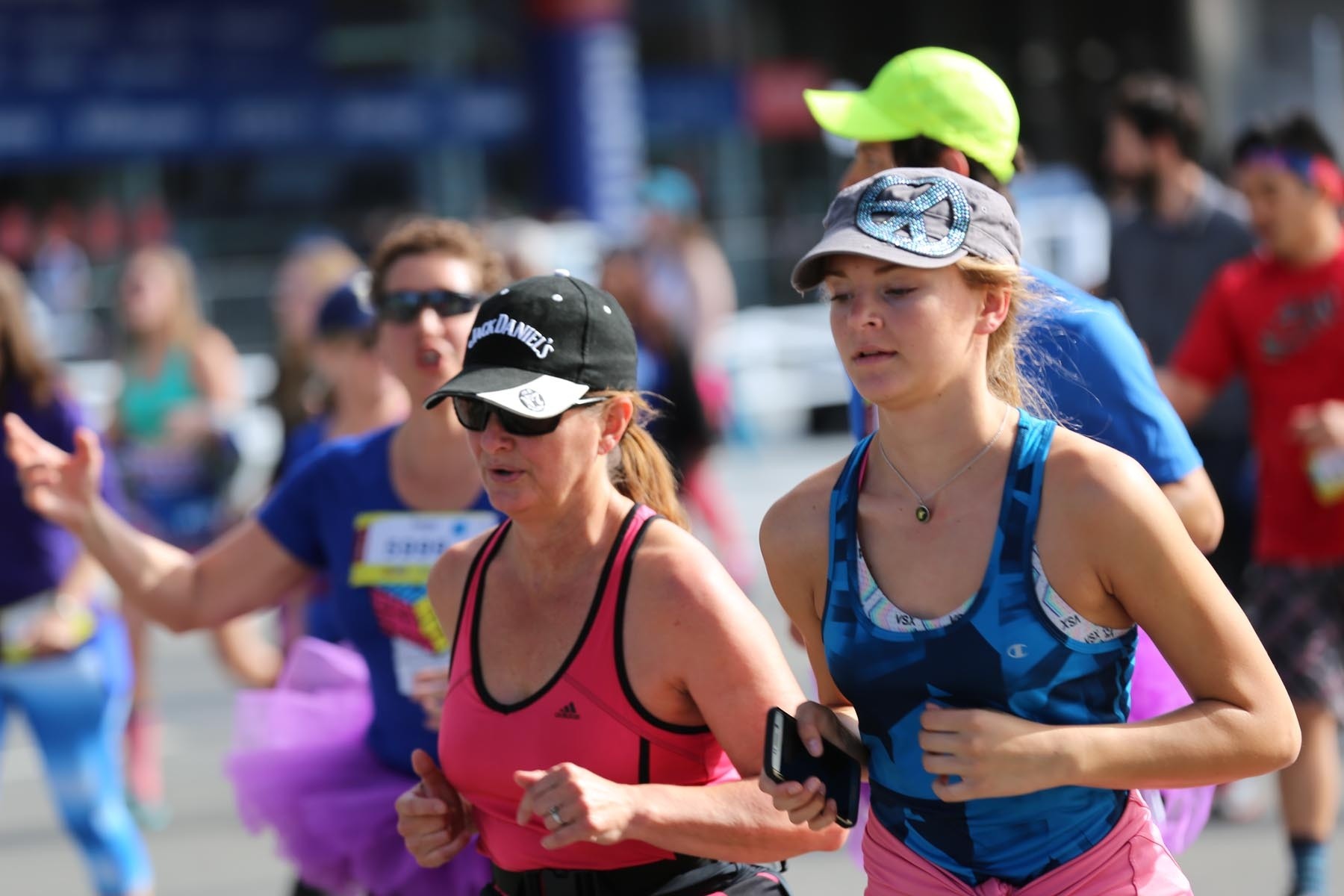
[880,403,1012,524]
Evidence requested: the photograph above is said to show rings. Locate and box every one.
[549,806,567,827]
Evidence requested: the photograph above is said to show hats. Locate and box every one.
[791,166,1022,294]
[803,46,1019,182]
[422,269,638,421]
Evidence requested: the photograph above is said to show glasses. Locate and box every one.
[452,394,609,435]
[377,287,486,320]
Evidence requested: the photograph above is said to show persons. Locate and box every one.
[393,269,850,896]
[0,40,1343,896]
[756,165,1303,895]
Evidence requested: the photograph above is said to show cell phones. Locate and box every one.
[765,706,862,827]
